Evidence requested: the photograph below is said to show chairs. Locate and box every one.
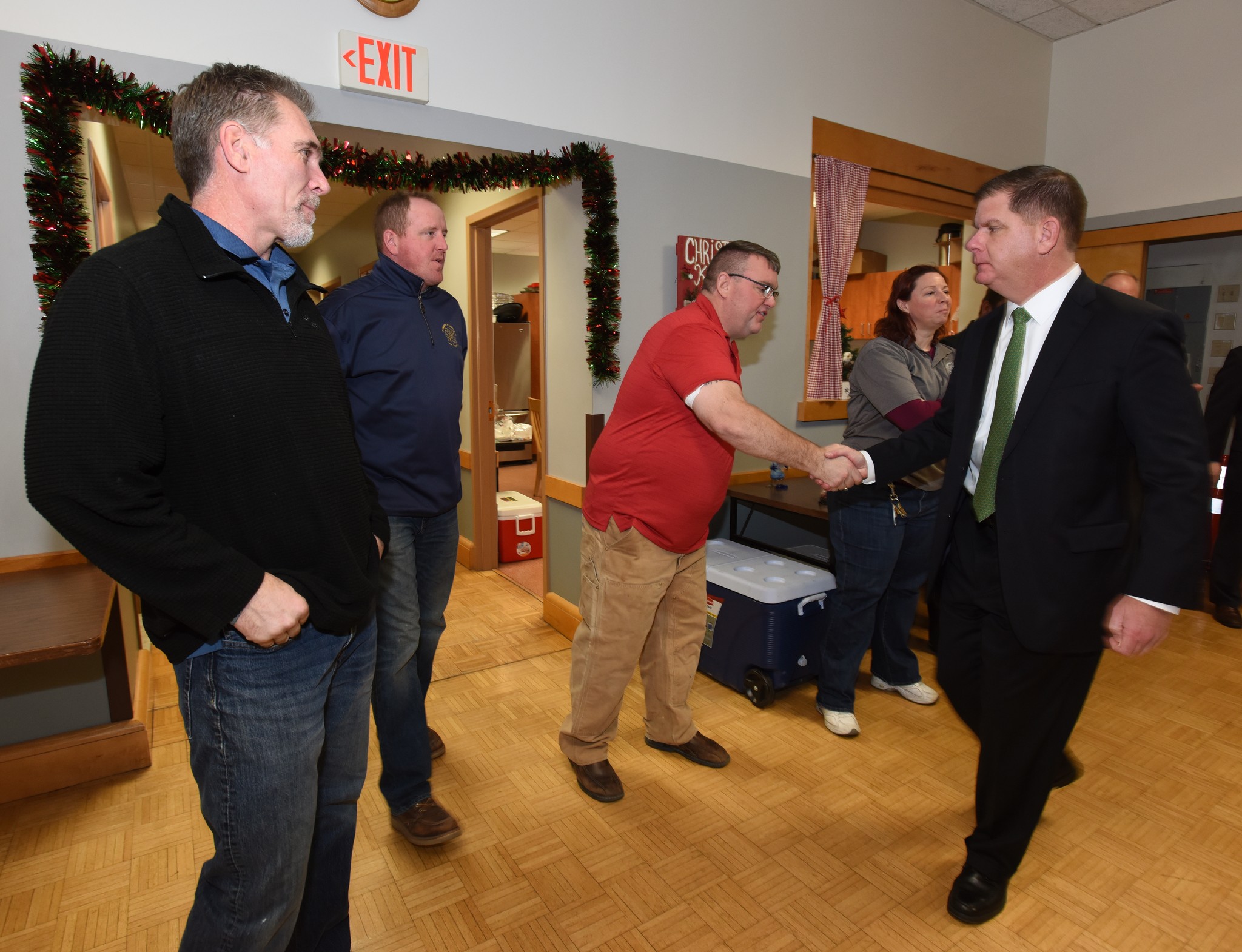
[526,395,543,497]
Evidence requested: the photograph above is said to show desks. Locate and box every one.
[494,437,535,468]
[730,476,936,654]
[0,559,135,743]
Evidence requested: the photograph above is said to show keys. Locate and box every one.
[887,500,908,528]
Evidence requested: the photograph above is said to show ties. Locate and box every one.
[970,307,1031,524]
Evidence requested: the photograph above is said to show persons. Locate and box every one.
[16,61,390,951]
[557,231,866,805]
[799,263,962,736]
[892,164,1214,930]
[287,188,476,849]
[1192,342,1241,638]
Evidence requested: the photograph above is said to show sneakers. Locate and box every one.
[870,675,940,705]
[815,701,861,735]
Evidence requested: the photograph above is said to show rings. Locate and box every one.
[844,487,848,492]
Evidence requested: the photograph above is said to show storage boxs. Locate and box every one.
[847,250,887,278]
[495,487,544,569]
[697,534,835,706]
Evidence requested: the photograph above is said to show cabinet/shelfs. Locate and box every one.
[513,293,541,400]
[812,261,960,341]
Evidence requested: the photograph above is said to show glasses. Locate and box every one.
[729,272,779,301]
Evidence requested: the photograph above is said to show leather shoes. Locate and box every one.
[947,866,1010,925]
[388,796,463,846]
[645,731,732,767]
[567,756,625,803]
[427,723,446,759]
[1212,604,1242,628]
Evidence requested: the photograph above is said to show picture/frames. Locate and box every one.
[1204,393,1211,413]
[1217,284,1240,302]
[1214,312,1237,330]
[1206,367,1222,383]
[1210,339,1234,357]
